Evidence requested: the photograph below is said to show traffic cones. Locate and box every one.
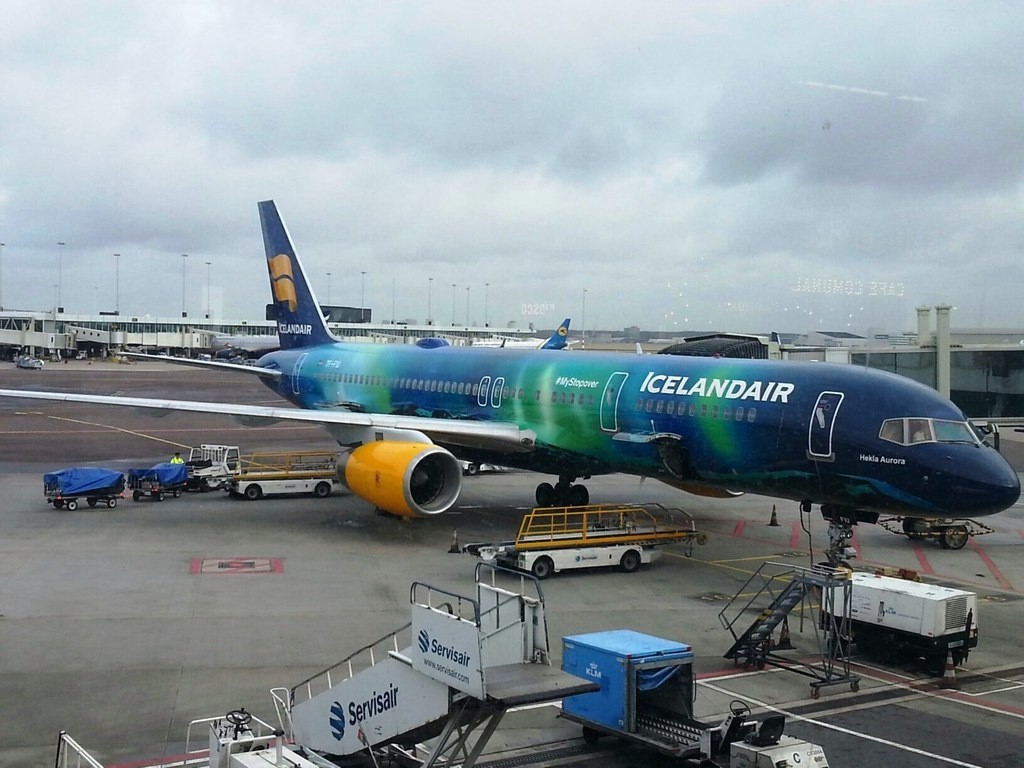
[766,504,781,526]
[775,615,797,650]
[940,650,961,689]
[447,527,461,554]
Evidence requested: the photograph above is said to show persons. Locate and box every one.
[170,452,184,464]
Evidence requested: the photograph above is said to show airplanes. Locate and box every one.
[0,198,1021,521]
